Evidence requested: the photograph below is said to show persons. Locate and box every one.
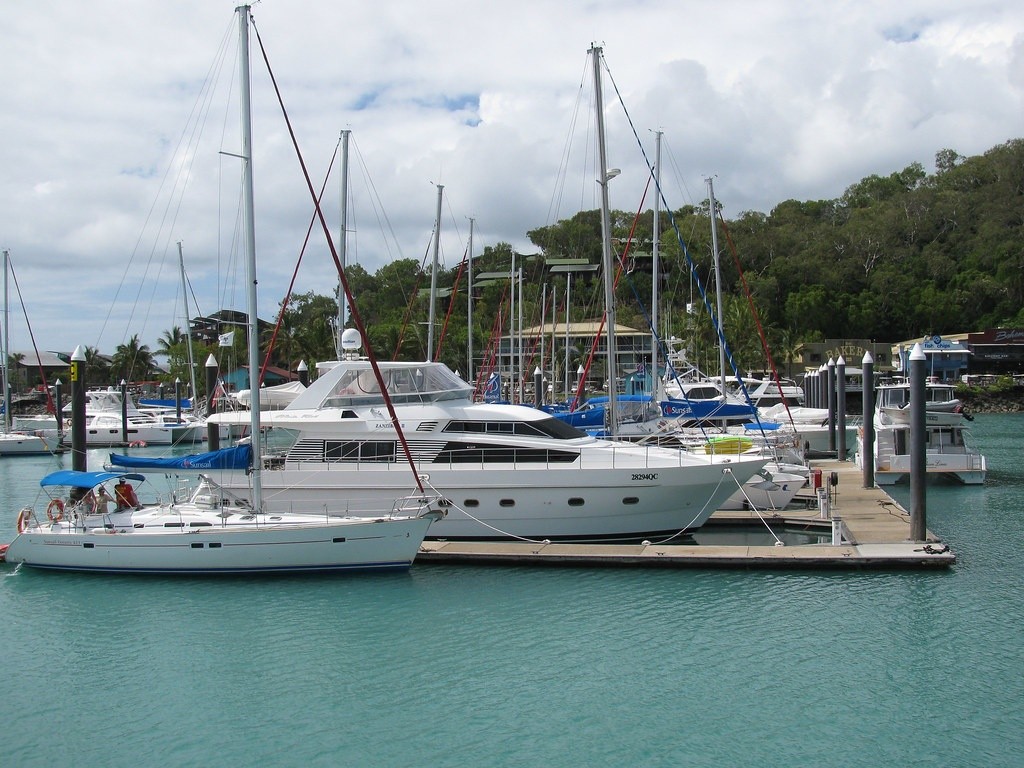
[69,486,98,518]
[95,487,116,512]
[115,477,140,509]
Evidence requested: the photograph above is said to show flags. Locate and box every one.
[637,356,646,378]
[482,374,501,403]
[212,381,225,408]
[219,330,234,346]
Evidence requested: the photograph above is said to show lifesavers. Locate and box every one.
[17,508,31,533]
[46,499,64,521]
[67,419,72,426]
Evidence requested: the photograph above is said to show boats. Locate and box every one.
[854,375,987,485]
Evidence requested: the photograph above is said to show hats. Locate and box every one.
[119,477,126,481]
[99,487,105,491]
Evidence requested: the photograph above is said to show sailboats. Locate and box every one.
[10,8,448,579]
[2,1,842,546]
